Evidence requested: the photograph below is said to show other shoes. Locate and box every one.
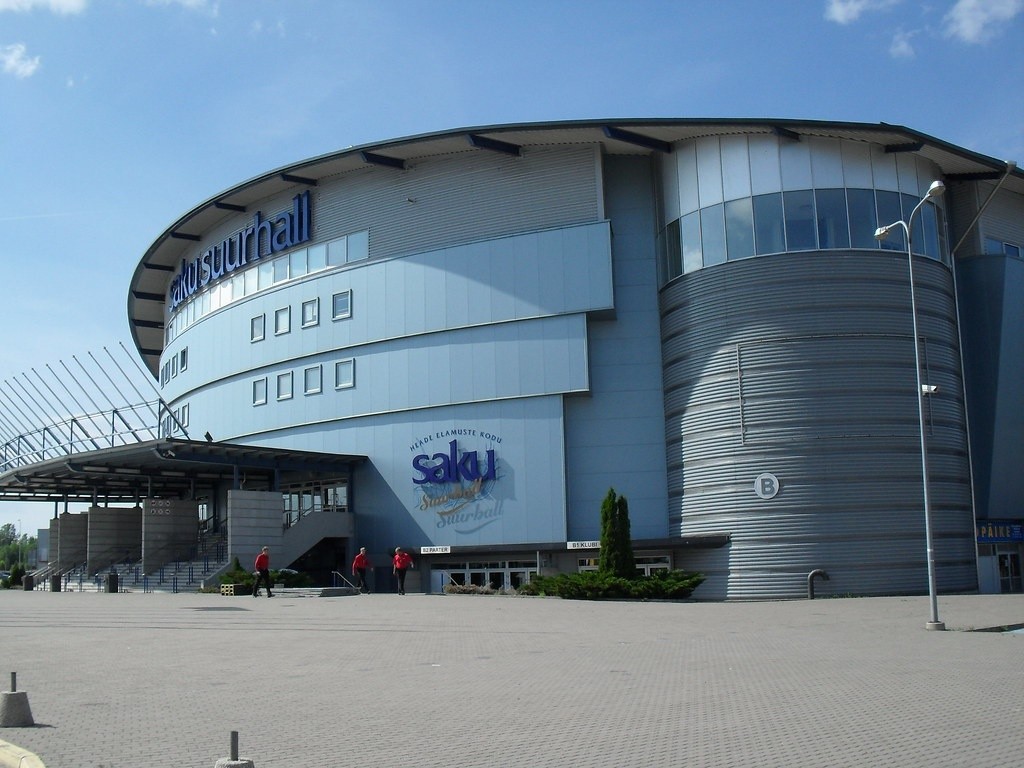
[398,591,405,595]
[253,594,258,598]
[268,595,275,597]
[368,590,370,594]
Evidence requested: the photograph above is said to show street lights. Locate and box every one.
[18,520,22,569]
[873,180,947,622]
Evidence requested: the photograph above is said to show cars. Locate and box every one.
[0,571,12,579]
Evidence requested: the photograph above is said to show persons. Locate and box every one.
[393,547,413,595]
[252,546,275,598]
[352,547,373,594]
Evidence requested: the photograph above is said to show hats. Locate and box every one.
[360,547,366,552]
[262,547,268,552]
[396,547,401,552]
